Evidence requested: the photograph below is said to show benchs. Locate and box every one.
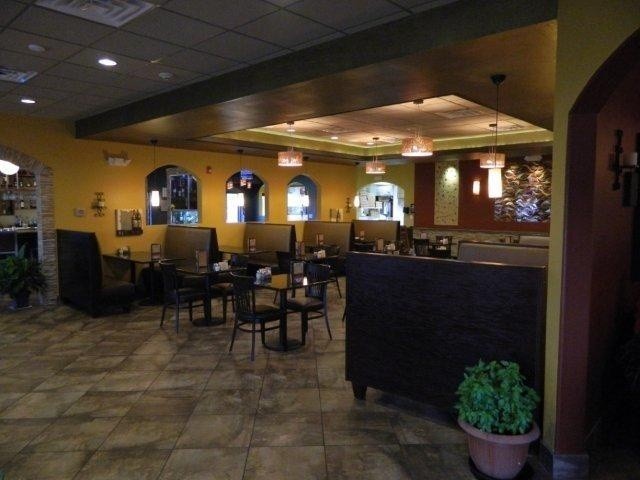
[229,223,296,274]
[346,236,549,419]
[142,225,238,305]
[57,229,135,316]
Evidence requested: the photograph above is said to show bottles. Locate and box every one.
[13,178,36,208]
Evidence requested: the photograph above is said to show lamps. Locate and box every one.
[277,121,303,166]
[480,73,506,169]
[401,98,434,156]
[365,137,386,174]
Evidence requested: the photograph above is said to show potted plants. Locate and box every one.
[0,244,48,308]
[452,358,542,480]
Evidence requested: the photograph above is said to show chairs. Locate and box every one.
[274,251,295,304]
[413,236,452,259]
[159,263,342,361]
[294,220,400,277]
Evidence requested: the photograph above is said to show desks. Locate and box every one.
[102,252,186,306]
